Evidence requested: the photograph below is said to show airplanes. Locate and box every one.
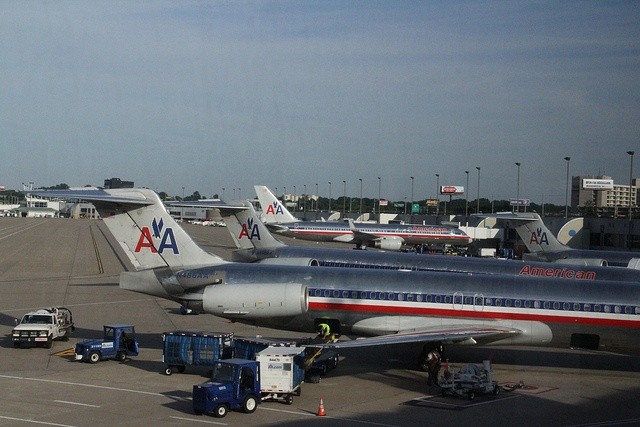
[471,210,640,269]
[253,184,473,251]
[161,198,639,282]
[11,187,640,371]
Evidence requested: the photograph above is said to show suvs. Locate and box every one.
[72,323,140,363]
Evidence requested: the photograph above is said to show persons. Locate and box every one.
[318,322,330,339]
[424,345,441,389]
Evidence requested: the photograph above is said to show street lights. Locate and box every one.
[359,178,362,212]
[342,180,346,213]
[293,185,296,211]
[475,165,481,213]
[304,184,306,211]
[515,161,521,213]
[377,176,381,213]
[315,183,319,209]
[283,185,286,207]
[464,170,469,218]
[410,176,414,216]
[434,173,439,214]
[563,156,571,220]
[626,150,635,222]
[328,181,332,212]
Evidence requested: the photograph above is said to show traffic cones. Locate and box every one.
[317,394,326,417]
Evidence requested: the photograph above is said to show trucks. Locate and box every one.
[161,329,307,417]
[11,304,75,348]
